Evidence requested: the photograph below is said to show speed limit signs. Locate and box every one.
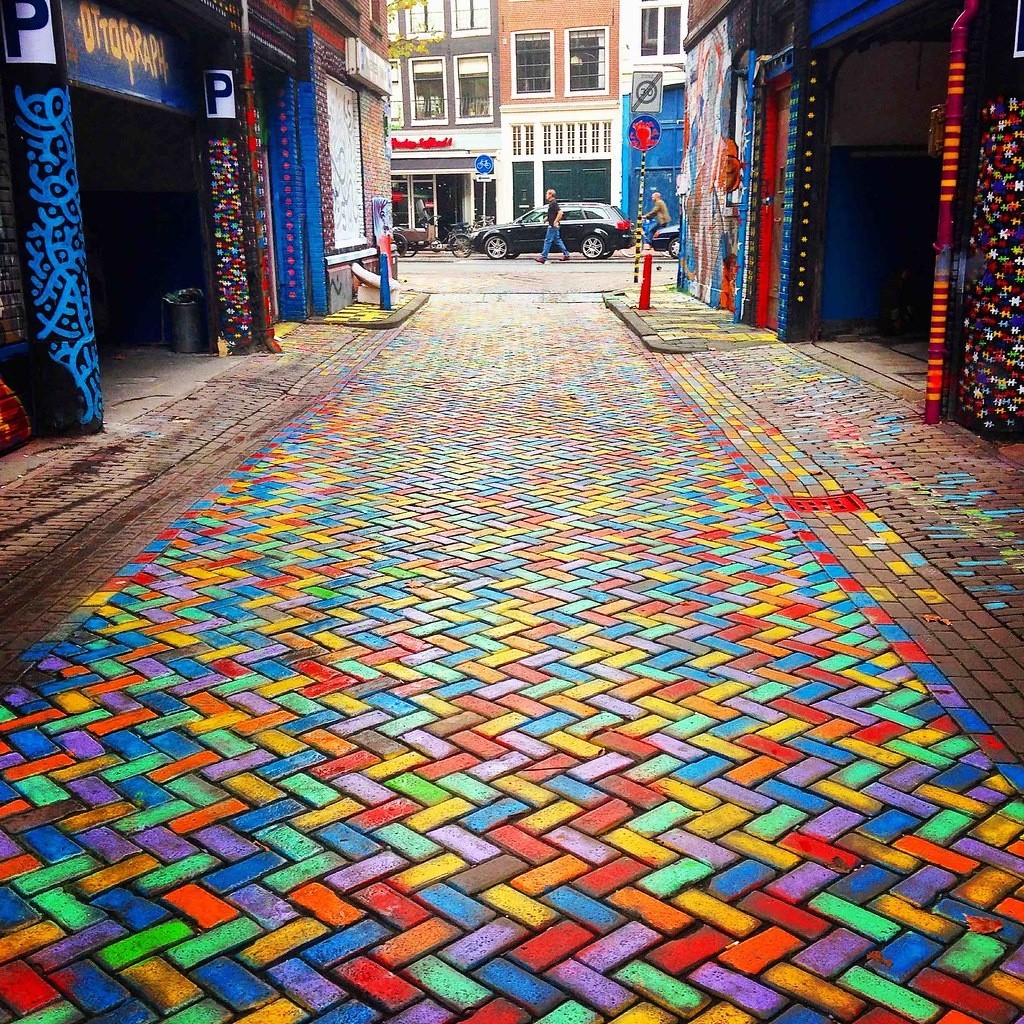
[631,70,663,113]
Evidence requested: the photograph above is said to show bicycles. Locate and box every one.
[449,216,497,255]
[619,216,674,258]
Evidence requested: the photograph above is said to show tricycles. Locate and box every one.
[393,198,473,258]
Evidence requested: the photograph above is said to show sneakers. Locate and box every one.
[560,256,570,261]
[535,258,545,264]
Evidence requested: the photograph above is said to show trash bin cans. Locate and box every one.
[163,287,210,354]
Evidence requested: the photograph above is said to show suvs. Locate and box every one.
[469,198,637,260]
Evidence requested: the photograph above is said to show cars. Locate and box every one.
[652,224,680,258]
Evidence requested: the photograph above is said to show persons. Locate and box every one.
[643,192,672,248]
[534,189,571,264]
